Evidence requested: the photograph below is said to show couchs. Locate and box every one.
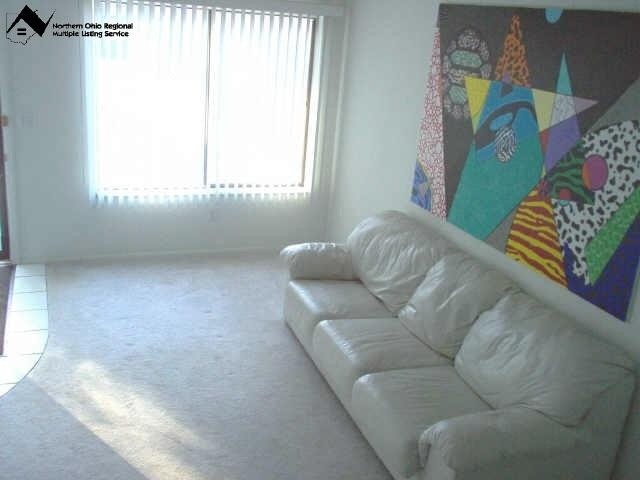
[279,211,638,479]
